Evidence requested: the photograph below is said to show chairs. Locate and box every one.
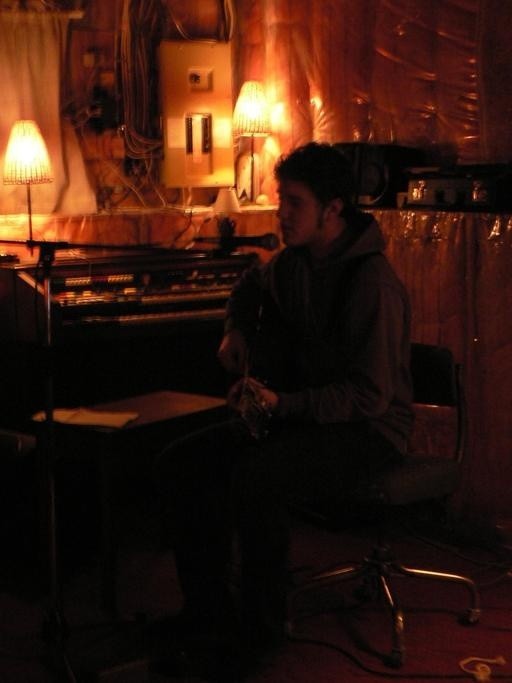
[282,345,484,667]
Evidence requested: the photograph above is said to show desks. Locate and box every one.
[28,388,230,676]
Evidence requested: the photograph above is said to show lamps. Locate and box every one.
[1,118,54,241]
[212,181,244,238]
[227,83,289,199]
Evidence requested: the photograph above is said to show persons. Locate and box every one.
[144,144,417,683]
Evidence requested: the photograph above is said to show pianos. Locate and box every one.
[1,249,262,348]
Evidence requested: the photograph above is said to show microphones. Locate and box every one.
[194,233,279,250]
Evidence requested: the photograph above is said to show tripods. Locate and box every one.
[0,260,133,683]
